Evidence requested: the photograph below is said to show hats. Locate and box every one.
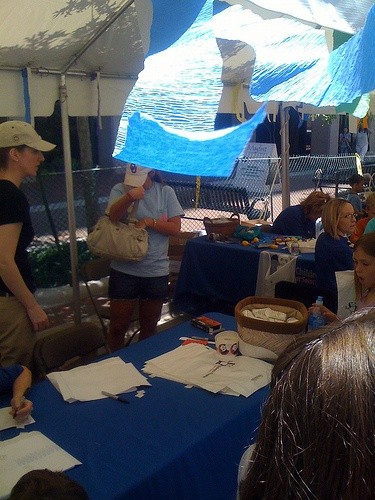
[0,120,56,151]
[125,162,153,188]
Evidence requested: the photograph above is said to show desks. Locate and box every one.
[173,233,316,318]
[0,314,315,500]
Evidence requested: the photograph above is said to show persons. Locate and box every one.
[348,191,375,240]
[339,127,352,154]
[236,306,375,500]
[10,469,87,500]
[0,364,35,420]
[355,124,371,163]
[308,233,375,320]
[347,175,368,218]
[105,161,184,351]
[271,191,330,234]
[0,120,58,374]
[315,198,357,316]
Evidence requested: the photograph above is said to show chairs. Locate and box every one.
[32,321,111,378]
[275,281,314,308]
[274,206,312,235]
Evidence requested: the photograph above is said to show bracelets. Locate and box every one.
[152,217,156,228]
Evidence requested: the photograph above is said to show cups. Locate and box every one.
[214,330,239,361]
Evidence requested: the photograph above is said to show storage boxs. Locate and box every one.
[234,296,310,356]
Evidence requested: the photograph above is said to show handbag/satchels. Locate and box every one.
[86,194,148,261]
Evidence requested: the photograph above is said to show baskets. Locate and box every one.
[204,213,240,240]
[235,295,308,356]
[246,198,270,221]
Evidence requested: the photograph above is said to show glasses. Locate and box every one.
[341,212,358,220]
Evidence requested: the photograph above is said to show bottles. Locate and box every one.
[291,239,299,255]
[308,296,325,334]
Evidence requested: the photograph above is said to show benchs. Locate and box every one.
[173,180,272,221]
[311,166,375,194]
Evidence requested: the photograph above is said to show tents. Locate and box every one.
[0,0,375,322]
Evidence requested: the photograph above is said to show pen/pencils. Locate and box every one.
[101,390,127,403]
[12,386,30,419]
